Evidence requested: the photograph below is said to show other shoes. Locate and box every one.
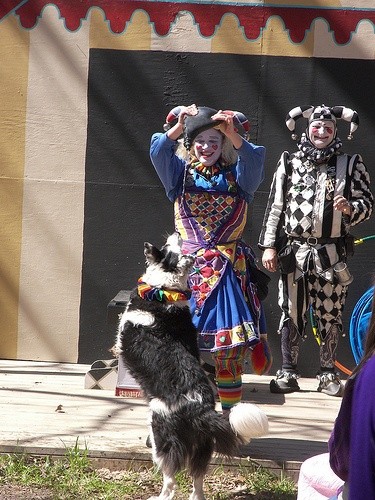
[270,370,301,393]
[316,373,345,396]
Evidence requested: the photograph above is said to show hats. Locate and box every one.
[285,104,360,141]
[163,105,250,151]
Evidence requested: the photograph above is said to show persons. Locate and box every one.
[258,103,374,396]
[328,297,375,500]
[145,104,273,448]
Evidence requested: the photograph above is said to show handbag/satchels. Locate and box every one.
[249,262,271,301]
[278,244,296,273]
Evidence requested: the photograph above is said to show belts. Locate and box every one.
[288,236,344,247]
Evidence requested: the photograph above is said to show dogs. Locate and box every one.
[114,230,270,500]
